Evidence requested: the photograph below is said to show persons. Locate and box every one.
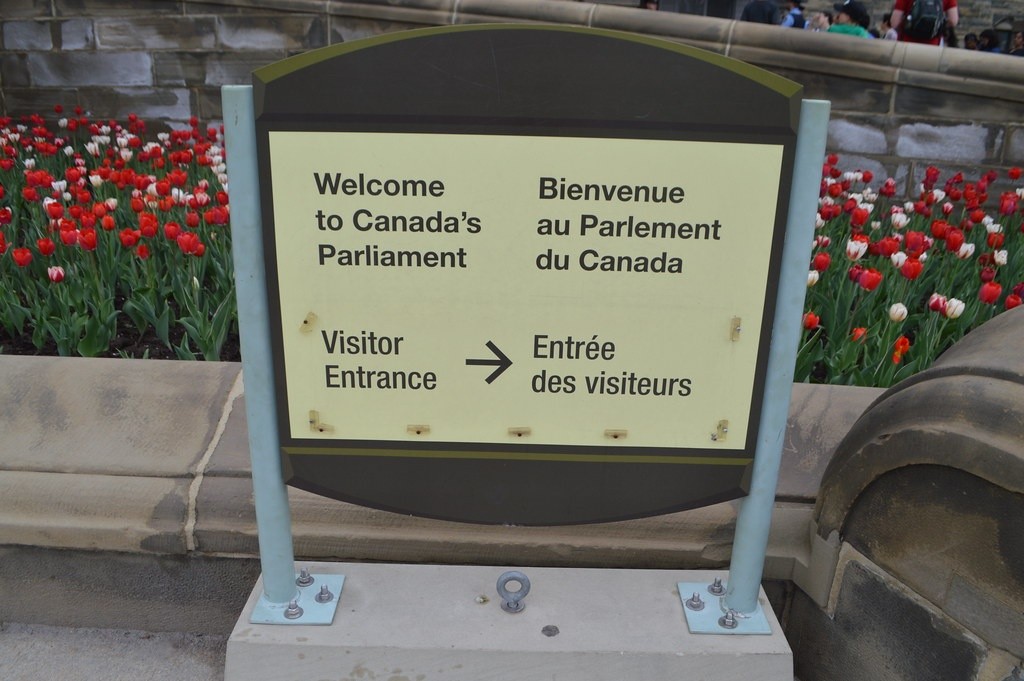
[740,0,1024,57]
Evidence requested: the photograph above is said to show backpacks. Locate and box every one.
[903,0,945,40]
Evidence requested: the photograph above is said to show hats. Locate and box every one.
[834,0,866,24]
